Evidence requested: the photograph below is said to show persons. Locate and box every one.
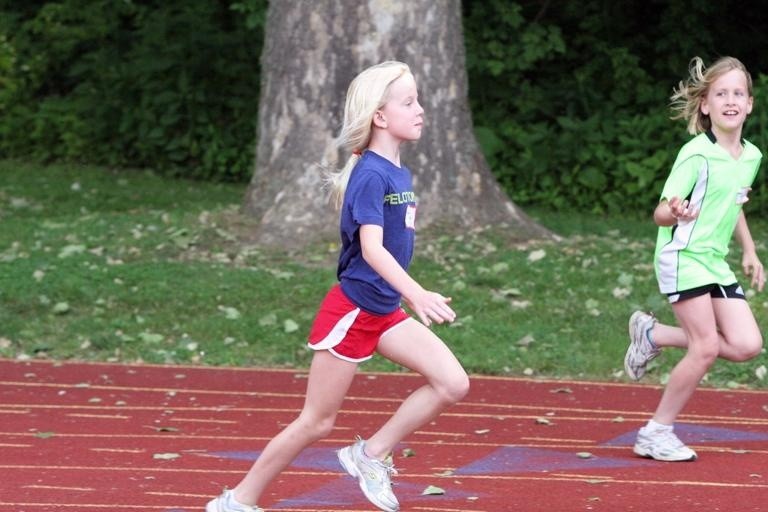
[203,60,473,511]
[623,55,766,462]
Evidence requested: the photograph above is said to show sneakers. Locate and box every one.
[624,310,662,381]
[633,427,698,463]
[206,488,265,512]
[337,439,401,512]
